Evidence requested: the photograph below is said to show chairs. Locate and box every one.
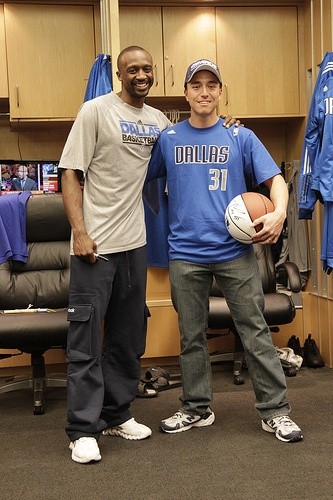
[0,193,71,417]
[170,243,302,384]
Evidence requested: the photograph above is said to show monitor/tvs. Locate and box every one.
[0,159,62,195]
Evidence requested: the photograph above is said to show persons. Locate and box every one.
[0,163,38,191]
[143,60,305,442]
[57,46,246,465]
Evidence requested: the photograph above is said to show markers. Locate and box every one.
[94,252,109,263]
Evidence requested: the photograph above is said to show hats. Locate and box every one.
[185,59,222,89]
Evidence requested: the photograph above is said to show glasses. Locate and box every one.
[17,170,27,174]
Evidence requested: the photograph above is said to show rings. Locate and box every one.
[268,238,272,242]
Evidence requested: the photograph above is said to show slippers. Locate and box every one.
[145,377,183,391]
[139,366,181,381]
[136,382,158,397]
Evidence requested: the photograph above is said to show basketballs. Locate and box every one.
[224,191,275,245]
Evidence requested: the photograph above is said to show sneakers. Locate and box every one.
[160,408,216,433]
[261,414,303,443]
[68,435,102,463]
[101,418,152,440]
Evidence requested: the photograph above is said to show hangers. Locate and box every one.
[163,108,180,125]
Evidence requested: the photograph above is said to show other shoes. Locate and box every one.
[304,334,324,367]
[288,335,303,360]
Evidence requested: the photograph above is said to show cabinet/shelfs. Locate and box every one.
[120,6,216,99]
[215,5,300,117]
[3,3,95,133]
